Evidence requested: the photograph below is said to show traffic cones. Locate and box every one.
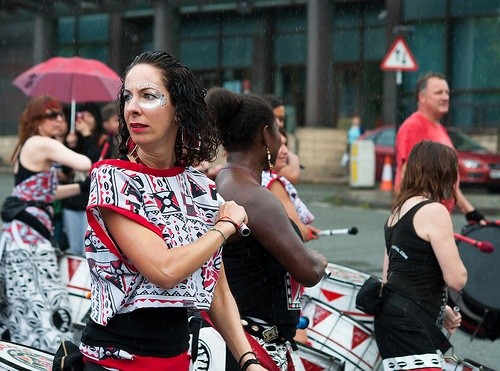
[379,156,393,192]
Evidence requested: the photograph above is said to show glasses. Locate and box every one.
[43,111,63,120]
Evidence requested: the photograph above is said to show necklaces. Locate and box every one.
[217,163,260,183]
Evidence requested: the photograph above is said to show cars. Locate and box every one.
[357,125,500,192]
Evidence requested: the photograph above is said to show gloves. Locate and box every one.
[465,209,485,224]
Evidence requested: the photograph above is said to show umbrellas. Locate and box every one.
[12,56,123,136]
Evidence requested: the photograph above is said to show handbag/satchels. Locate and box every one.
[355,274,389,315]
[51,340,85,371]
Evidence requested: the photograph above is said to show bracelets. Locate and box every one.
[242,359,260,371]
[239,352,256,365]
[215,216,239,234]
[210,229,226,245]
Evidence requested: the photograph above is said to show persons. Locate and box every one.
[191,99,320,242]
[204,87,328,343]
[347,116,361,154]
[0,95,92,355]
[52,103,123,214]
[375,140,467,371]
[382,72,490,284]
[79,50,274,371]
